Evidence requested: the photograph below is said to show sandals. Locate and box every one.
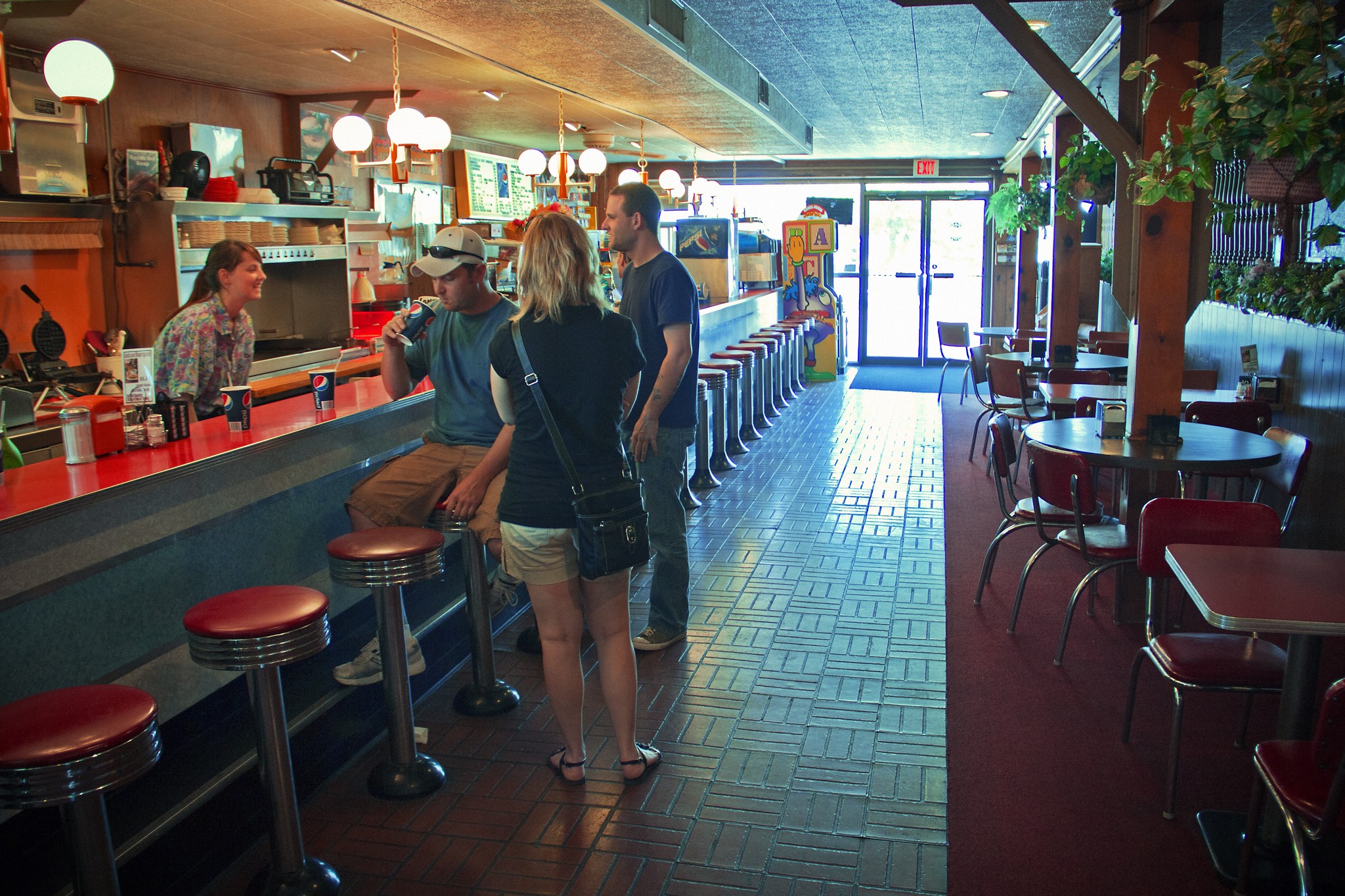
[620,740,660,784]
[548,745,588,785]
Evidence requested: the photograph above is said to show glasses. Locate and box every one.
[422,243,483,261]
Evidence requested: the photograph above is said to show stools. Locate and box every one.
[326,525,444,802]
[679,315,819,511]
[2,683,164,894]
[183,585,339,896]
[435,495,523,719]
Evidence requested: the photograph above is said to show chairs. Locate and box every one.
[1005,438,1140,668]
[1124,495,1303,820]
[1192,676,1345,896]
[935,321,1275,515]
[1249,426,1312,534]
[973,412,1104,617]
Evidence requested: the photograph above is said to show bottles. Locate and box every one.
[1235,382,1245,398]
[1244,380,1252,399]
[147,415,167,445]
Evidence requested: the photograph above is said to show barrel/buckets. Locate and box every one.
[96,355,125,397]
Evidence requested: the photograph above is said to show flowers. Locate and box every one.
[1206,256,1345,333]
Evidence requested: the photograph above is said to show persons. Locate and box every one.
[473,209,663,783]
[328,226,526,686]
[138,238,267,430]
[582,180,701,652]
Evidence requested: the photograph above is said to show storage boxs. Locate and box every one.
[171,123,246,187]
[487,257,512,282]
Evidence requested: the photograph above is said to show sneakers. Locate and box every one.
[332,639,425,686]
[632,623,688,650]
[464,566,521,621]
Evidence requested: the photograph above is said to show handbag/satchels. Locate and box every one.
[572,481,651,581]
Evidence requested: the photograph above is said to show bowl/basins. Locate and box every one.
[159,187,189,201]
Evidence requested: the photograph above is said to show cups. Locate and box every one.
[60,407,97,464]
[395,300,436,346]
[678,226,719,254]
[695,282,710,299]
[220,385,251,432]
[308,370,336,411]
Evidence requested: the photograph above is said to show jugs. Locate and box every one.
[377,261,405,285]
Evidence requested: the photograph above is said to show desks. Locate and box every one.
[1162,541,1344,883]
[1025,417,1282,628]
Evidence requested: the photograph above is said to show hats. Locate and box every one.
[410,224,488,277]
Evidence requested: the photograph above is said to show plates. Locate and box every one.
[177,220,345,249]
[202,176,239,202]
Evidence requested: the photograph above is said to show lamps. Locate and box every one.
[517,91,607,199]
[328,47,582,132]
[666,144,720,211]
[0,0,115,154]
[616,119,680,204]
[330,26,453,185]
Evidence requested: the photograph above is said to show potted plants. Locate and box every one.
[1049,86,1116,221]
[999,141,1051,239]
[1122,0,1345,254]
